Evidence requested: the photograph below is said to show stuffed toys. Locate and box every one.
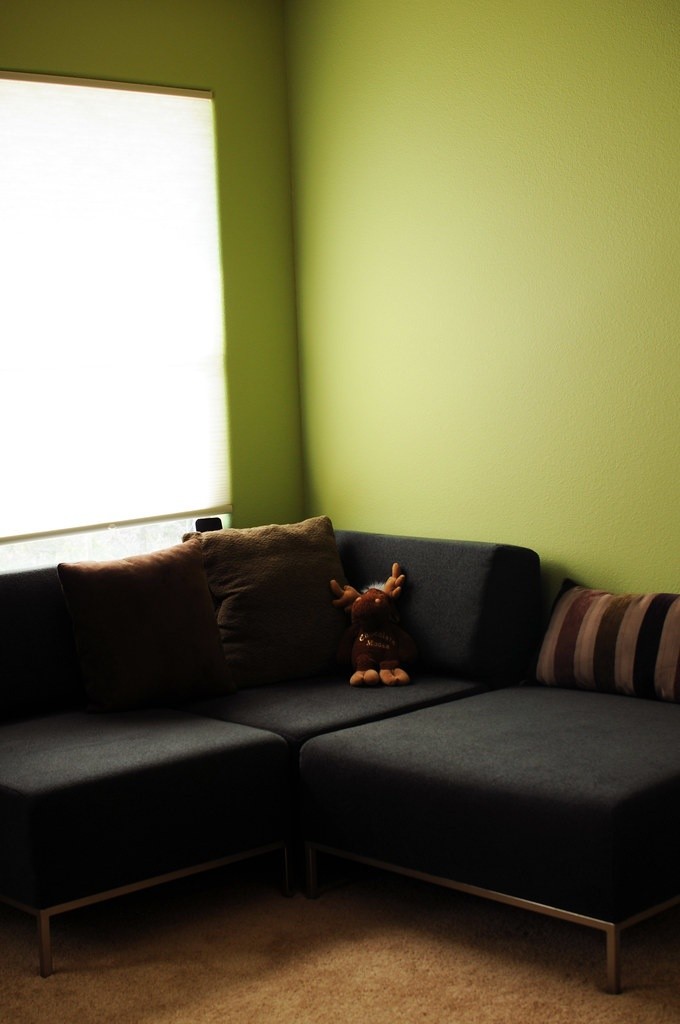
[329,563,410,685]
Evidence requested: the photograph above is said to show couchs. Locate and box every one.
[0,516,680,994]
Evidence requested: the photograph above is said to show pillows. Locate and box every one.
[57,535,237,714]
[181,515,350,689]
[525,579,680,703]
